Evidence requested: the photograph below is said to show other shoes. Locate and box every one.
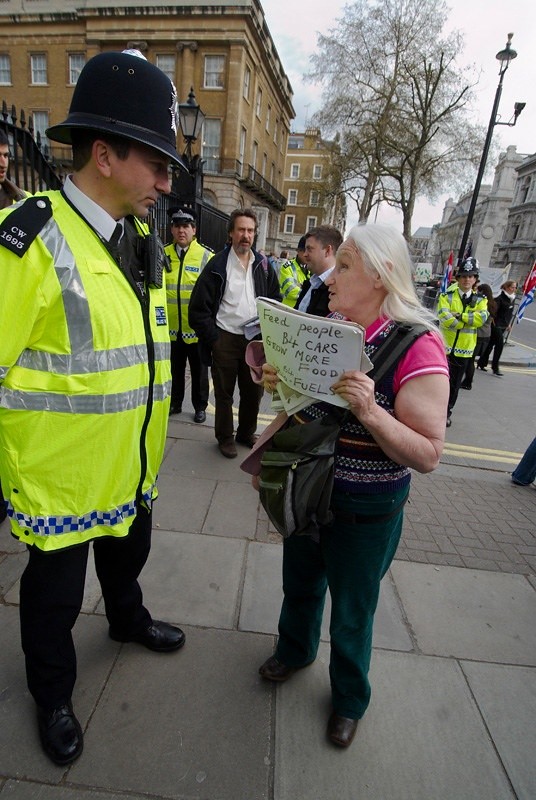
[446,411,453,427]
[460,382,472,390]
[219,434,237,458]
[235,430,257,448]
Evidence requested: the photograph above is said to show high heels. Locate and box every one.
[493,369,503,375]
[477,363,488,371]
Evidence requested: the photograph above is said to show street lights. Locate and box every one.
[456,33,526,267]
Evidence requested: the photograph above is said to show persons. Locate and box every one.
[258,221,448,747]
[258,247,289,274]
[188,210,283,458]
[293,226,344,318]
[0,50,184,765]
[0,129,33,211]
[280,235,311,308]
[161,206,217,423]
[436,257,536,486]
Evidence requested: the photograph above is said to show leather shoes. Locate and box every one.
[194,411,206,423]
[109,619,185,652]
[327,708,359,748]
[36,694,83,766]
[258,653,314,680]
[169,407,182,415]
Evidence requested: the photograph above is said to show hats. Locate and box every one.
[167,207,197,224]
[456,259,477,277]
[45,52,189,175]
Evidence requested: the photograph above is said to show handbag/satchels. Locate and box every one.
[258,417,342,544]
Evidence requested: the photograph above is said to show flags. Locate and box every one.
[517,259,536,324]
[435,245,455,297]
